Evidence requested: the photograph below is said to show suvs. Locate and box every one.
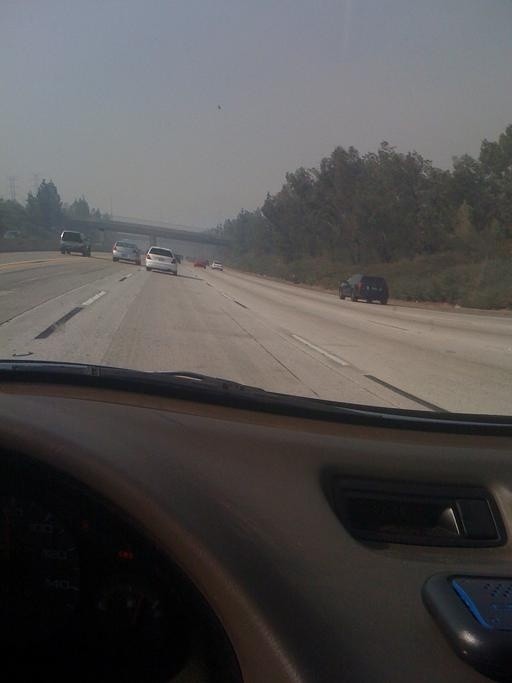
[59,229,92,257]
[338,272,388,304]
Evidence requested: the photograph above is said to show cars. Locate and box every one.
[145,245,177,275]
[175,253,224,272]
[111,238,141,266]
